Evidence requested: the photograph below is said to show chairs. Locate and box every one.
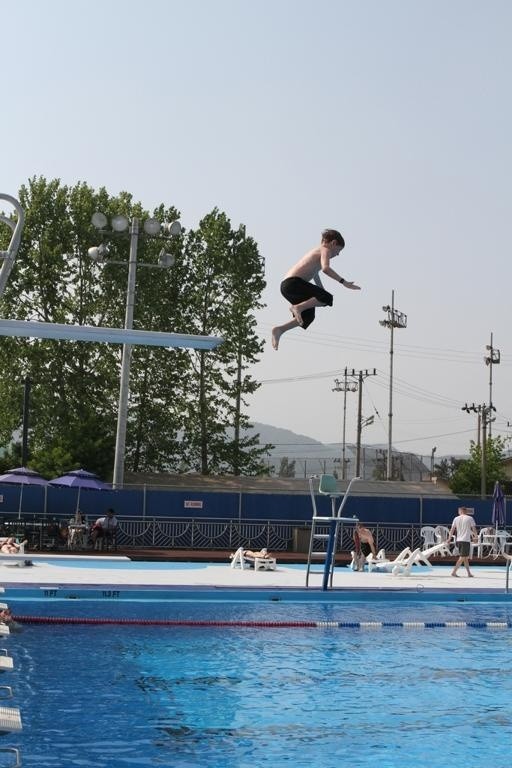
[348,542,446,577]
[231,547,275,572]
[420,525,511,560]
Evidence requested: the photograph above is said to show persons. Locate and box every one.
[447,507,478,577]
[270,231,360,350]
[87,509,117,550]
[1,536,28,554]
[356,524,378,570]
[230,549,273,559]
[68,510,87,544]
[353,522,364,571]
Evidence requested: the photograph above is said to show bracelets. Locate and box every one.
[339,279,344,284]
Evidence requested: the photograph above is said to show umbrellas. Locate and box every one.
[48,469,115,525]
[0,467,52,519]
[491,481,506,532]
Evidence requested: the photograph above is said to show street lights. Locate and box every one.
[356,415,376,479]
[86,211,182,493]
